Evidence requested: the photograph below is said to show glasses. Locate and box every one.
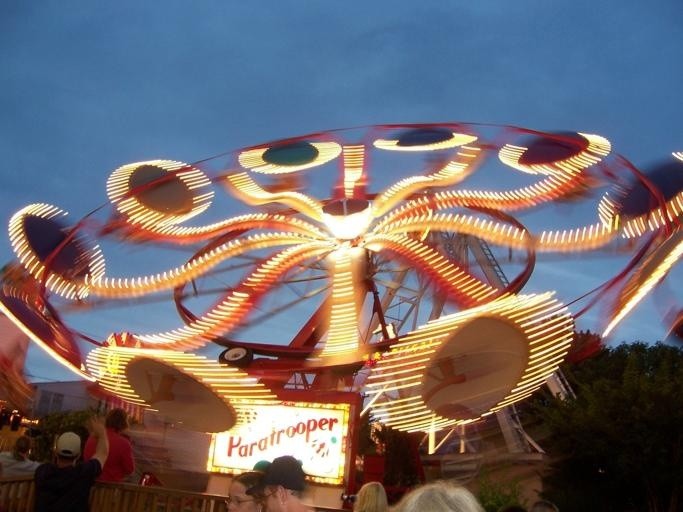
[223,497,253,503]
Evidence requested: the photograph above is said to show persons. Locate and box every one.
[138,472,151,486]
[230,455,315,512]
[391,480,487,512]
[83,406,135,486]
[0,435,39,481]
[0,416,25,450]
[350,481,390,511]
[32,414,109,512]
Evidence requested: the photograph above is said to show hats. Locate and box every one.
[55,432,80,456]
[245,455,304,494]
